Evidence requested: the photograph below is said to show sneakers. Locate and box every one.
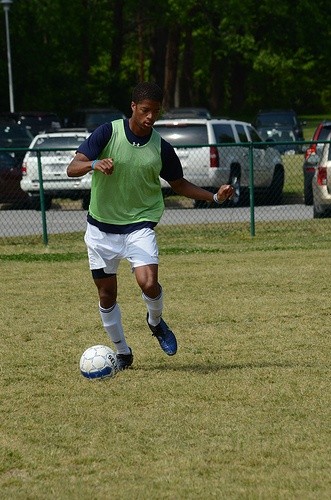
[145,312,177,357]
[112,346,134,370]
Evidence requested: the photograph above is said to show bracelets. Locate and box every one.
[213,193,227,204]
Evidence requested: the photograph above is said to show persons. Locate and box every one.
[66,82,235,378]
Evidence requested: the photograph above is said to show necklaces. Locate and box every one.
[91,160,99,170]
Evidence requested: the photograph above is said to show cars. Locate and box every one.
[302,118,331,219]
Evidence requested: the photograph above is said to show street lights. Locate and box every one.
[0,0,16,114]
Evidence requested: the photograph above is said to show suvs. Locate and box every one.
[152,108,284,208]
[251,108,308,154]
[0,110,128,211]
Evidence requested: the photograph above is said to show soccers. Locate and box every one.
[78,345,121,381]
